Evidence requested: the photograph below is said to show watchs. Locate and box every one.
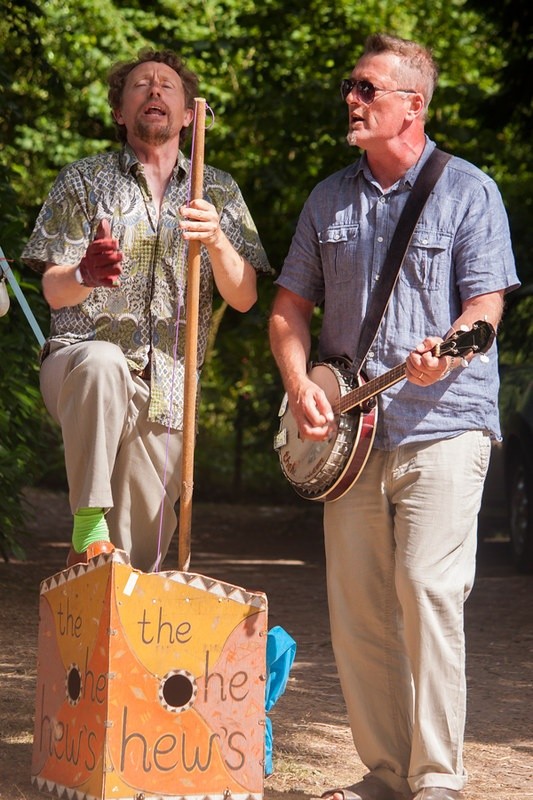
[439,356,455,381]
[74,258,100,287]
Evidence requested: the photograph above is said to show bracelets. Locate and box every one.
[81,257,105,286]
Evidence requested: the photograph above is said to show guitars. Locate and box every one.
[275,315,497,503]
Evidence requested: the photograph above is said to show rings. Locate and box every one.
[418,372,424,379]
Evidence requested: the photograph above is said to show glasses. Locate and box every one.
[340,78,417,104]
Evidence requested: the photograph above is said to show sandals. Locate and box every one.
[320,778,405,800]
[411,786,461,800]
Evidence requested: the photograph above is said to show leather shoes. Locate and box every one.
[65,539,117,567]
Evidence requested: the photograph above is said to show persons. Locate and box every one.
[268,32,523,800]
[20,45,270,573]
